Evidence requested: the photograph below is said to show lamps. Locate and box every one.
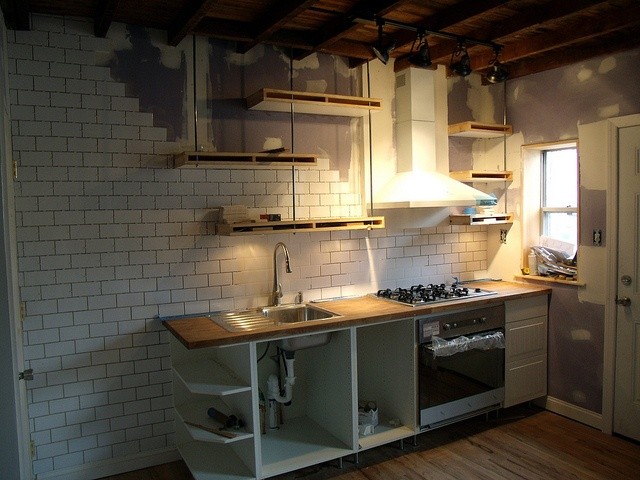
[373,28,395,63]
[484,59,510,83]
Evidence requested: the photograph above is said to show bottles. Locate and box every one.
[527,249,538,275]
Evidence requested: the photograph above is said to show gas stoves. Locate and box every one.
[367,283,498,307]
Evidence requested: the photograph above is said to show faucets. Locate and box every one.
[272,242,293,306]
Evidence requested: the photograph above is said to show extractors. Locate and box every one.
[368,68,497,210]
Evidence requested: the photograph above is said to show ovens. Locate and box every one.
[417,302,506,429]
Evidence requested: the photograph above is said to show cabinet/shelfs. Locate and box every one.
[449,121,515,227]
[167,315,418,479]
[507,294,547,407]
[174,86,385,236]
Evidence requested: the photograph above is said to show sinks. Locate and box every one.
[258,303,334,324]
